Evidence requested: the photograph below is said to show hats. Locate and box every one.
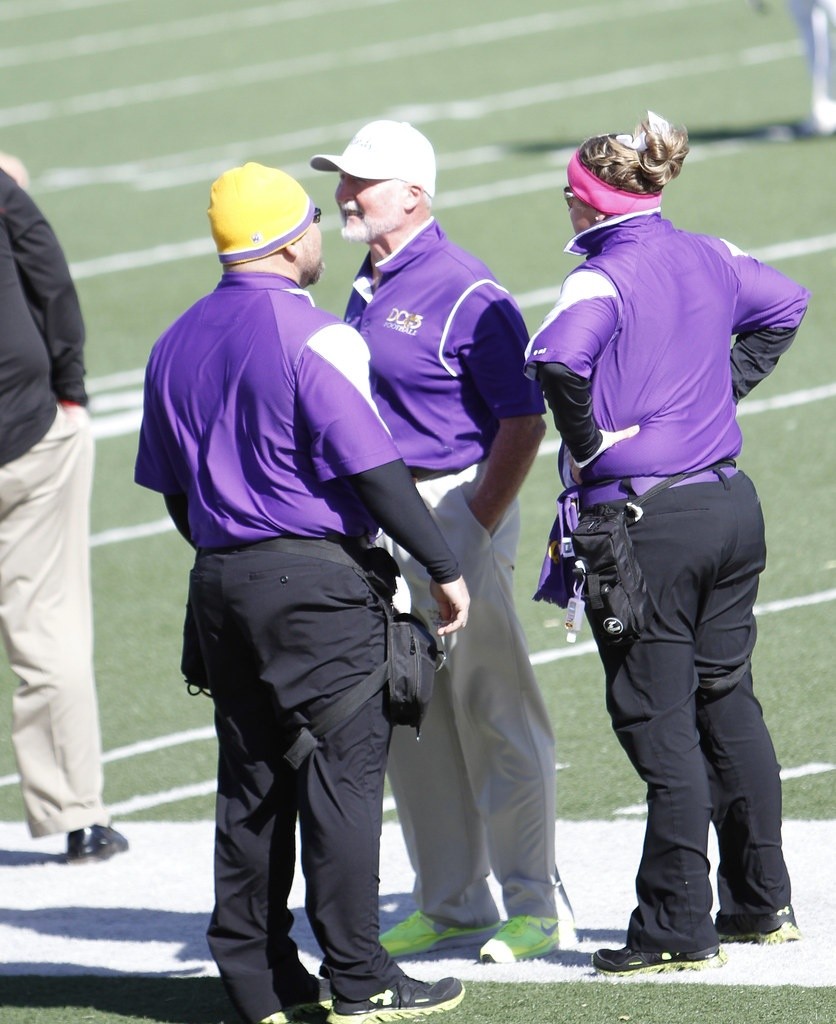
[207,161,315,265]
[310,119,436,199]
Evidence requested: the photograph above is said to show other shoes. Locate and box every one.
[65,826,129,865]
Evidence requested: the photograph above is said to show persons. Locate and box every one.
[133,162,470,1024]
[789,0,836,135]
[524,108,810,978]
[0,152,130,865]
[309,120,578,965]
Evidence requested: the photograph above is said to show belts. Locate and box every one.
[405,465,442,483]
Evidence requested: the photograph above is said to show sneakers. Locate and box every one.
[478,914,560,965]
[714,904,803,945]
[591,944,730,978]
[325,974,466,1024]
[379,909,504,959]
[257,977,333,1024]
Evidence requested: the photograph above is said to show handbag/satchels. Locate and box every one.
[358,614,442,727]
[570,509,655,638]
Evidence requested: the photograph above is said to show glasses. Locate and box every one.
[563,186,576,208]
[313,207,322,224]
